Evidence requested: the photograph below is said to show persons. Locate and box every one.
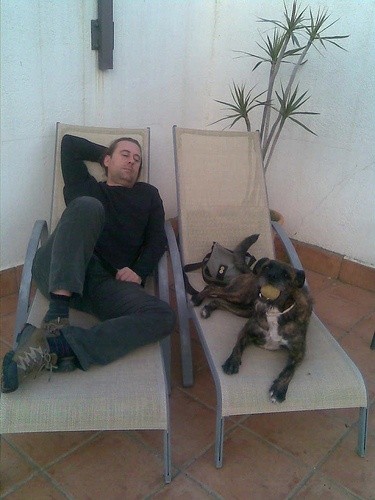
[0,134,177,394]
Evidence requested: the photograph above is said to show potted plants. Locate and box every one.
[203,2,351,243]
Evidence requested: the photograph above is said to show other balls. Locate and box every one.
[261,284,280,300]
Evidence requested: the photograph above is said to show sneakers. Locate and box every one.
[2,323,59,394]
[41,310,71,334]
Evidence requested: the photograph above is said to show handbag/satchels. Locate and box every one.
[183,241,256,297]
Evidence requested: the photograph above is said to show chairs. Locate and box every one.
[0,122,173,484]
[163,126,368,468]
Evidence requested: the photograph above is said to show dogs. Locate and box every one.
[188,233,314,404]
